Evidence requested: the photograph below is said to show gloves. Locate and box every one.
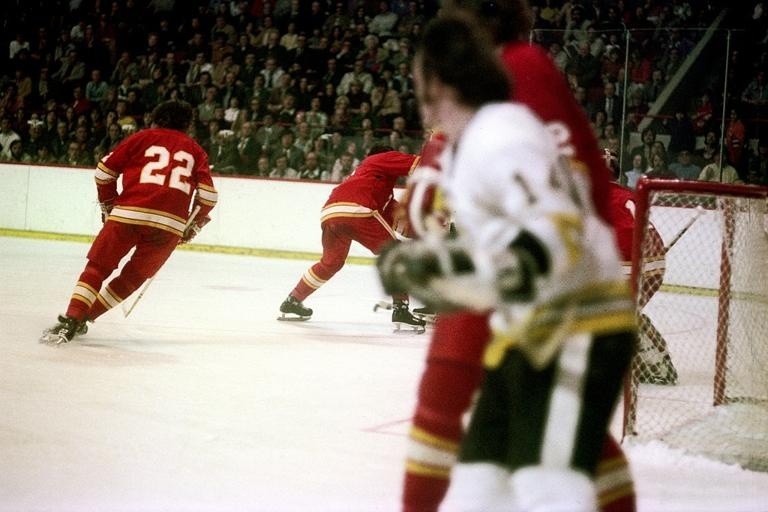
[176,215,211,245]
[98,193,121,222]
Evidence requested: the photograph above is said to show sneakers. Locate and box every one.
[49,316,89,342]
[640,362,678,380]
[279,293,314,314]
[392,304,434,326]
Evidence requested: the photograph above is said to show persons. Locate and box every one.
[3,0,767,186]
[36,98,218,342]
[278,143,426,335]
[377,1,680,511]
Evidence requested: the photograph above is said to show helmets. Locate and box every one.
[151,100,193,131]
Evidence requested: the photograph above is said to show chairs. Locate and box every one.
[1,0,768,192]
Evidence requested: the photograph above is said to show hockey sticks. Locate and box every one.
[123,206,201,317]
